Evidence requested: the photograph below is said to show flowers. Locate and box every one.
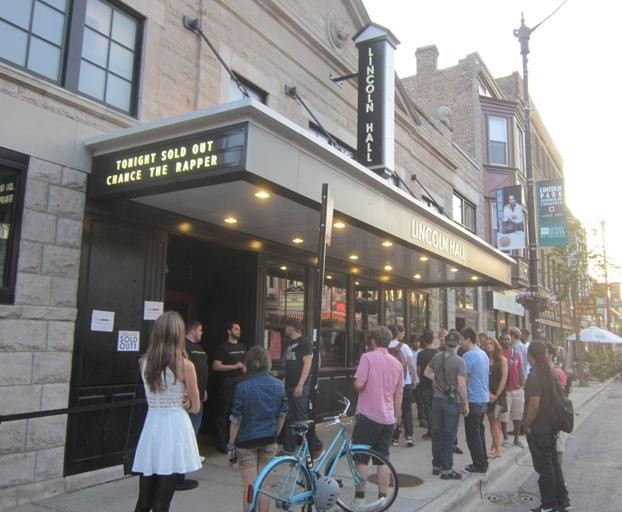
[515,291,556,313]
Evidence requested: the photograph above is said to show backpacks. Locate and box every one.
[387,342,409,381]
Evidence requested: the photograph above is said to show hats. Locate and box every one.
[286,319,306,331]
[444,329,461,347]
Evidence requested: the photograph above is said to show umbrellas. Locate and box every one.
[565,325,622,354]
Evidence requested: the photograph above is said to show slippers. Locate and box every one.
[503,439,511,448]
[486,448,502,459]
[512,439,525,449]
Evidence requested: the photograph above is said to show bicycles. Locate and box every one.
[246,390,398,511]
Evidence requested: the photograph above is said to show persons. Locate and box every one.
[348,325,405,512]
[211,320,247,452]
[503,193,524,233]
[520,340,574,512]
[129,310,202,511]
[226,346,290,512]
[275,317,325,462]
[181,318,209,464]
[386,323,571,479]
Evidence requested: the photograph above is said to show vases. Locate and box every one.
[522,301,544,314]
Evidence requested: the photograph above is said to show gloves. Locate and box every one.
[227,442,239,464]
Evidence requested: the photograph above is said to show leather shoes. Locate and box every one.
[175,478,201,489]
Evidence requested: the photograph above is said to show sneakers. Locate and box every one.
[462,462,489,475]
[439,470,465,482]
[432,464,442,476]
[527,501,571,512]
[310,445,326,462]
[422,432,432,440]
[506,430,524,436]
[405,435,417,447]
[391,437,400,447]
[419,419,429,428]
[454,447,463,455]
[274,449,295,458]
[348,496,366,511]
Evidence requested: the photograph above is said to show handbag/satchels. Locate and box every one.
[557,396,574,435]
[119,435,147,477]
